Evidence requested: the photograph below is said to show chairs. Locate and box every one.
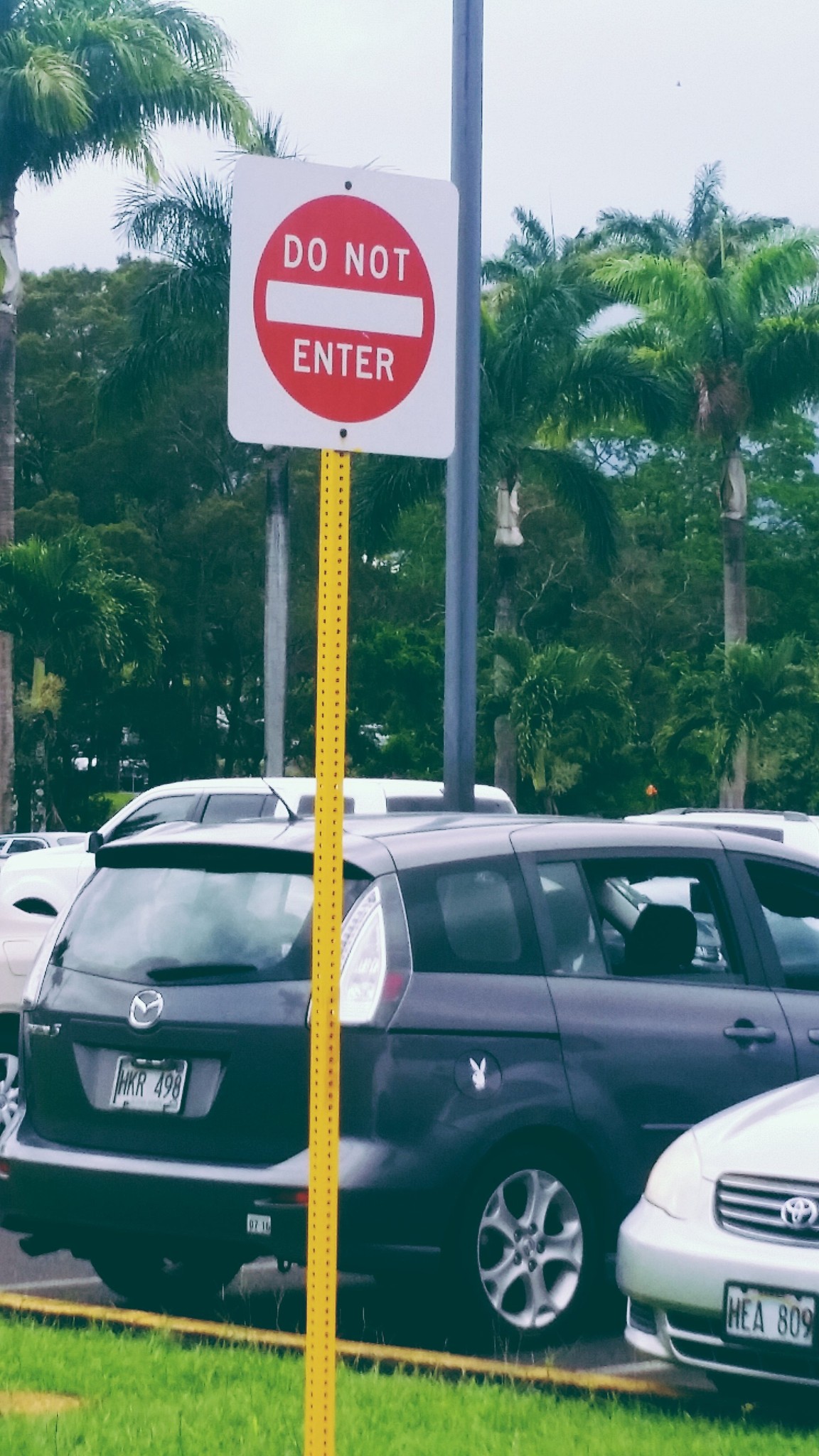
[623,903,698,979]
[544,889,590,976]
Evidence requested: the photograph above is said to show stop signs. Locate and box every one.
[228,151,464,458]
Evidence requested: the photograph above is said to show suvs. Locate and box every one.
[3,779,517,1027]
[1,767,819,1351]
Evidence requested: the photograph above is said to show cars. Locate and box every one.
[615,1075,819,1405]
